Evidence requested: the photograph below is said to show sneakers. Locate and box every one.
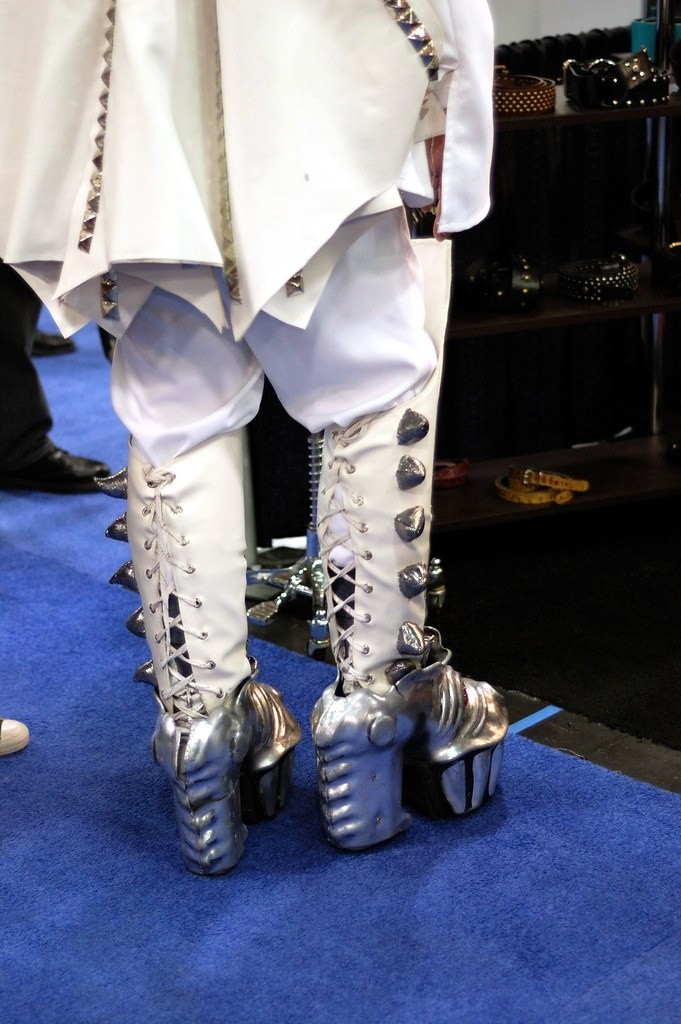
[0,718,29,756]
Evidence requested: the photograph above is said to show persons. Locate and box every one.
[0,256,111,495]
[0,1,508,875]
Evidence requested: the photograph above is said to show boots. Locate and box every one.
[310,238,509,848]
[94,427,302,875]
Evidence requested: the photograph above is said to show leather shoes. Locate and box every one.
[0,449,110,492]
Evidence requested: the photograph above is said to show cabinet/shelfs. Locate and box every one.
[243,22,681,657]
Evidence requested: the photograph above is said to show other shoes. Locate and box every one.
[29,330,77,356]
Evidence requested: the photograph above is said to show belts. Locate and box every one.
[433,43,673,507]
